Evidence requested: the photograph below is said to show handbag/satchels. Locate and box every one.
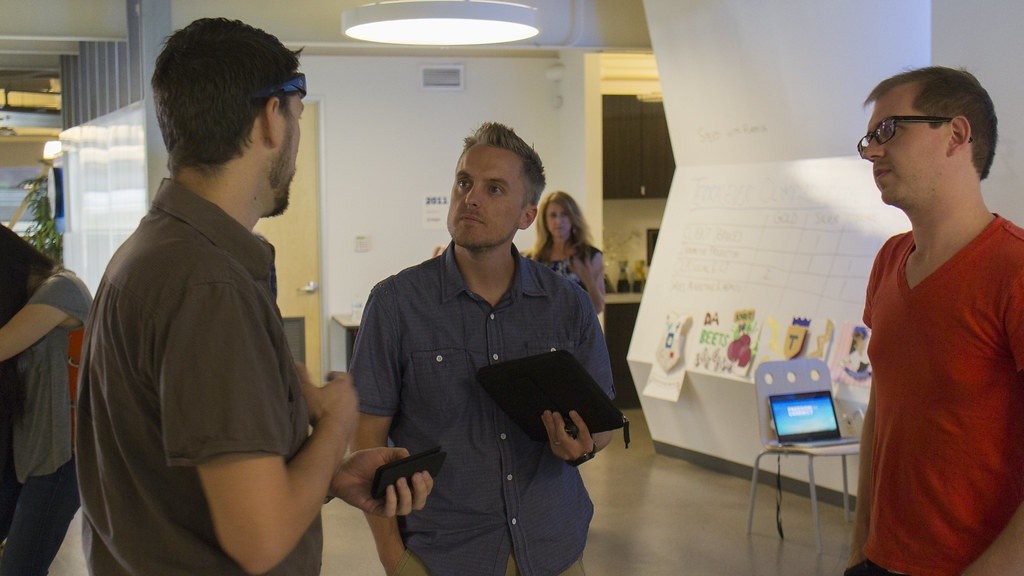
[477,350,630,448]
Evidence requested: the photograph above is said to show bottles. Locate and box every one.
[632,268,645,293]
[617,267,631,294]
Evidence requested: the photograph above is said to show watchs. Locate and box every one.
[565,444,598,468]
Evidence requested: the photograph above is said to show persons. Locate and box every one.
[520,191,606,314]
[74,19,435,576]
[0,224,93,576]
[345,119,614,576]
[843,65,1024,576]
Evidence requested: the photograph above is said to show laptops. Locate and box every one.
[769,390,861,448]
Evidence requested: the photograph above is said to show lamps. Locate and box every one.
[340,2,538,46]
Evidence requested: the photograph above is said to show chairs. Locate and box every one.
[746,358,862,552]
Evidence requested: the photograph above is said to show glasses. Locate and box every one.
[252,73,308,101]
[857,115,974,158]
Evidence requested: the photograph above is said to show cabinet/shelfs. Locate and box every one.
[602,94,675,198]
[605,302,642,410]
